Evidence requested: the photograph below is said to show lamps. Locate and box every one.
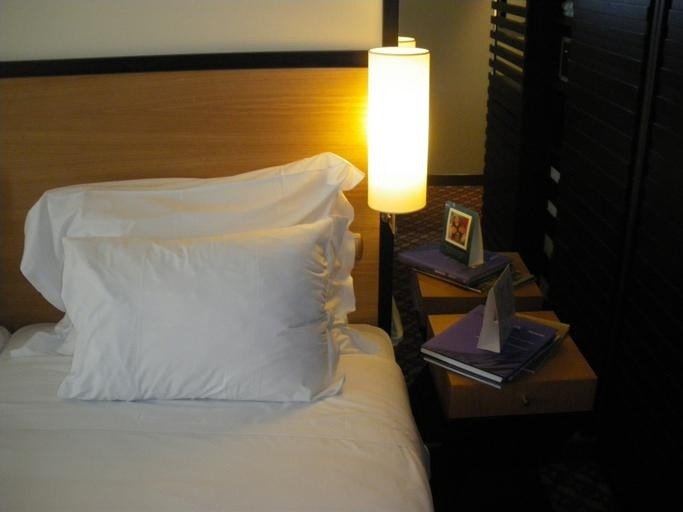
[368,47,432,215]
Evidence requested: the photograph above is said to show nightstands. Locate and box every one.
[426,310,598,464]
[411,251,543,342]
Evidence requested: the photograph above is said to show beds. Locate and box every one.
[0,51,431,512]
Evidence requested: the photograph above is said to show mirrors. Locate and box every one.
[391,0,655,396]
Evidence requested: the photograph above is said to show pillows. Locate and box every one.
[57,212,353,403]
[18,151,369,355]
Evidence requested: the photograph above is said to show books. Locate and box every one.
[416,303,571,390]
[393,242,537,296]
